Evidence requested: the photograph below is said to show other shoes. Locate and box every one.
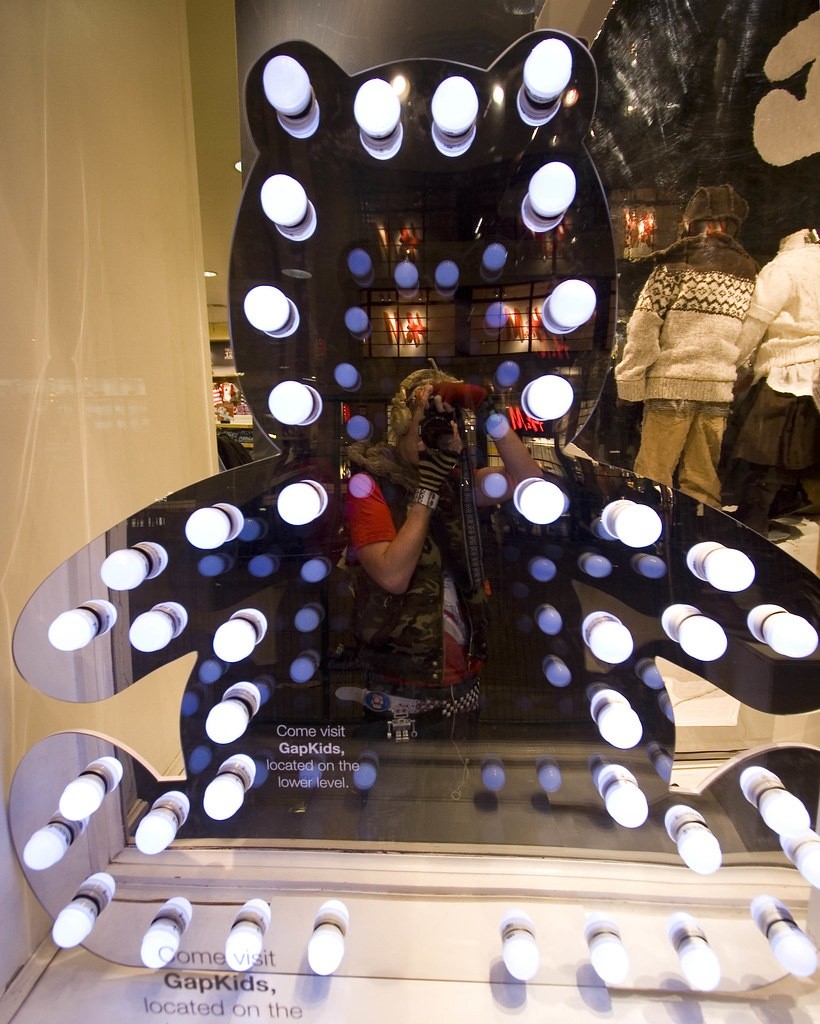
[721,494,761,522]
[768,483,813,520]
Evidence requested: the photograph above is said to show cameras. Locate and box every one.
[418,396,464,451]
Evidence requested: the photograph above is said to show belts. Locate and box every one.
[334,677,480,716]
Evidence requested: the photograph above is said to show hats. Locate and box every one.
[683,184,750,237]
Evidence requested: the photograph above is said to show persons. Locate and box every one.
[614,187,761,511]
[734,190,820,535]
[342,366,545,743]
[212,378,241,416]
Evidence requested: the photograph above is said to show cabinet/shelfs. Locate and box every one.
[208,321,254,448]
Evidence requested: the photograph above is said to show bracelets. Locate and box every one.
[413,489,439,510]
